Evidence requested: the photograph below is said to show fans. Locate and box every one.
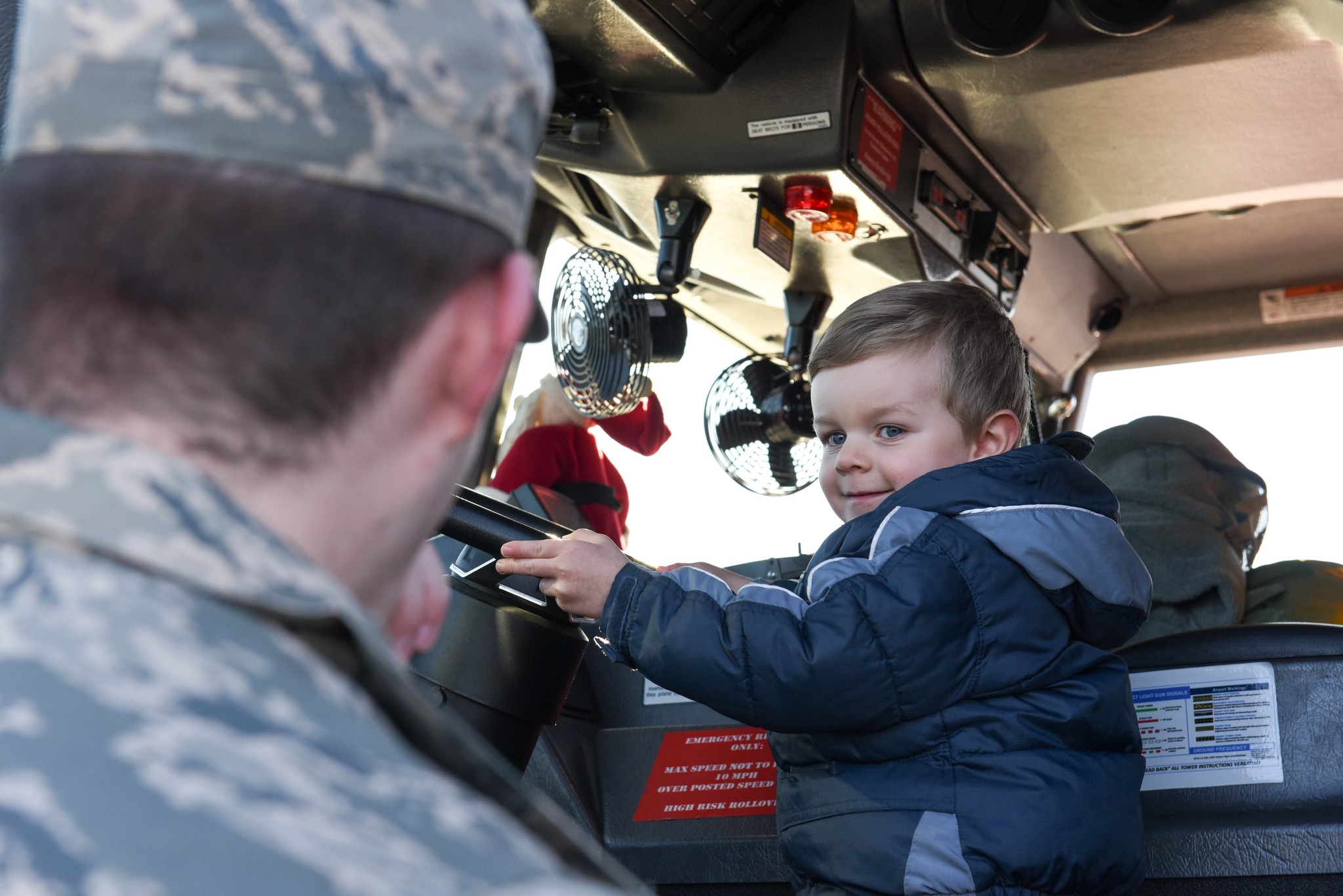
[549,174,713,421]
[701,289,840,496]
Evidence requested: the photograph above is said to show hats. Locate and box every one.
[4,1,556,252]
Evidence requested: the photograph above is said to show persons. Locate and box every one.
[0,4,629,896]
[494,280,1158,896]
